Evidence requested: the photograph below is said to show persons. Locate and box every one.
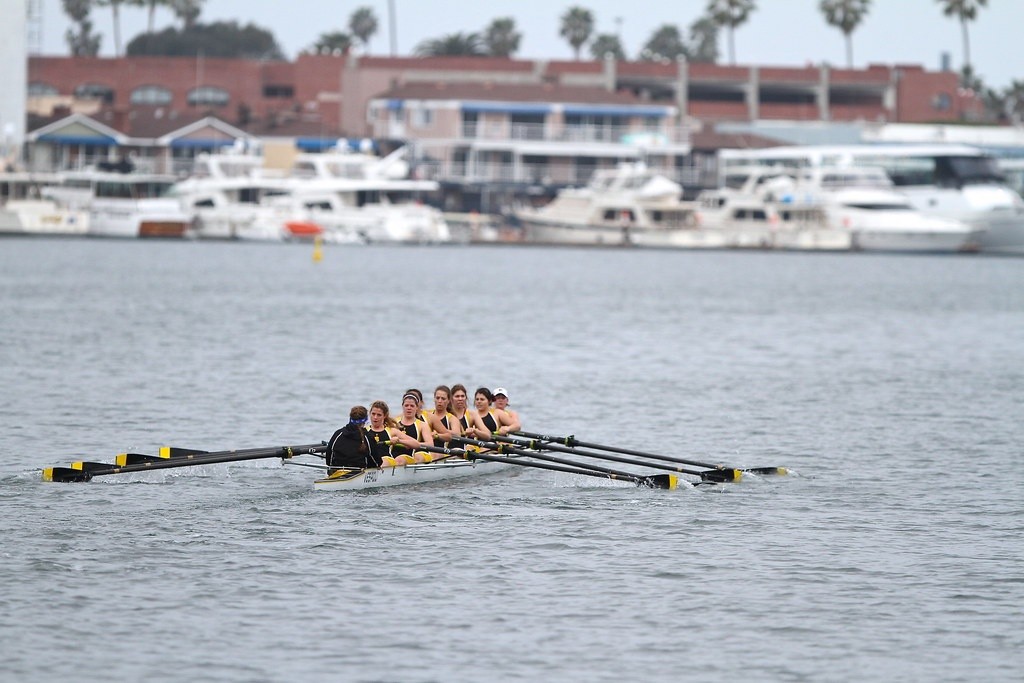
[430,384,520,460]
[365,401,420,468]
[395,389,451,462]
[391,393,435,465]
[326,406,383,476]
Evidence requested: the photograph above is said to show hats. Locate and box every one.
[493,388,509,398]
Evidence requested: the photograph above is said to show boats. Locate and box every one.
[312,452,546,491]
[0,154,1024,257]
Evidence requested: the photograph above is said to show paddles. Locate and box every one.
[394,428,790,491]
[43,440,329,483]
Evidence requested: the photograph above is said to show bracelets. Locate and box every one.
[435,434,438,439]
